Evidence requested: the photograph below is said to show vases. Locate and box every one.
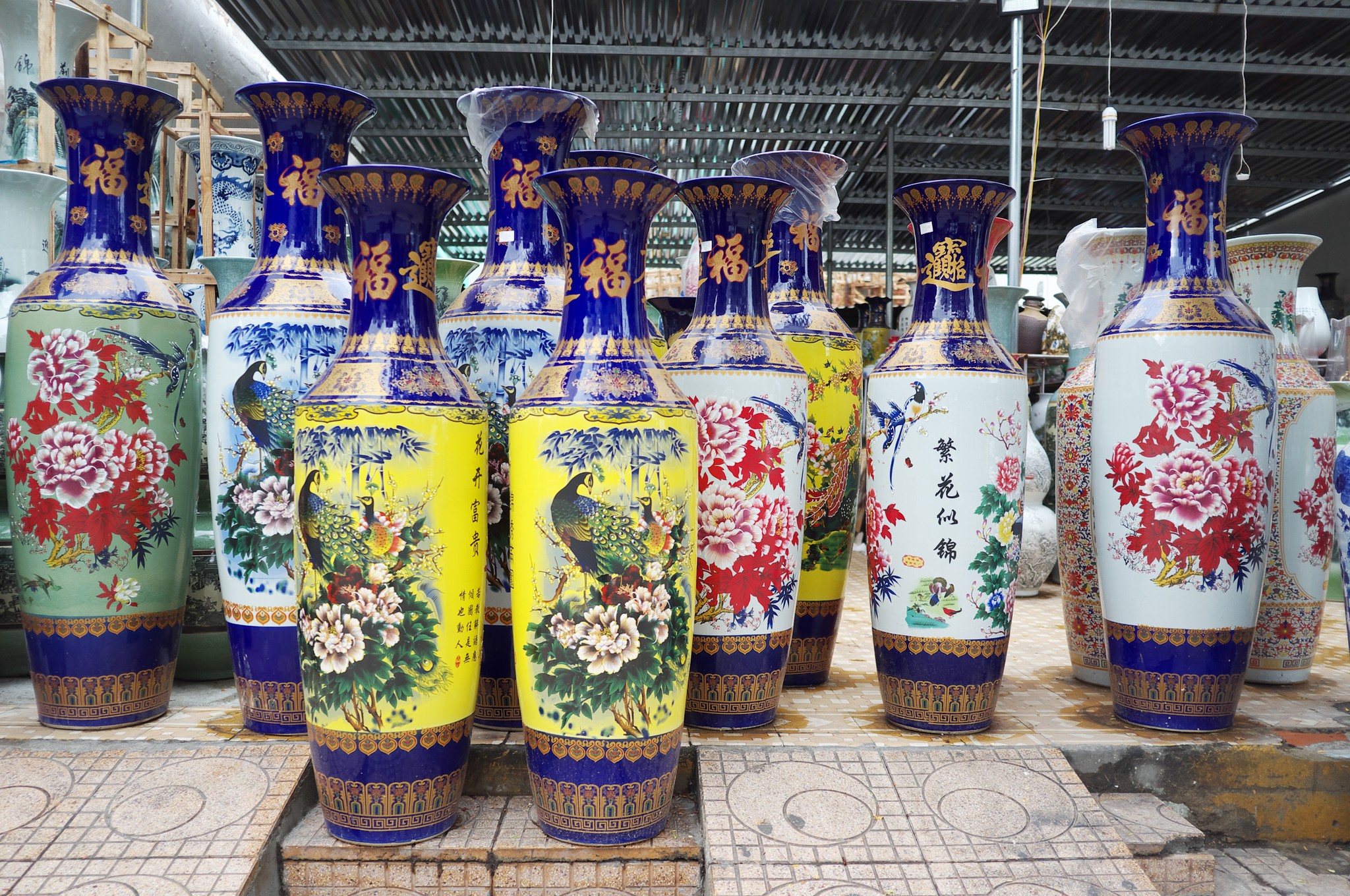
[1049,217,1149,691]
[685,171,811,732]
[903,209,1016,296]
[197,246,258,305]
[4,74,203,735]
[433,253,483,322]
[204,76,379,724]
[868,163,1034,733]
[731,145,863,691]
[985,280,1026,358]
[288,161,485,847]
[1086,103,1290,741]
[898,277,917,338]
[860,290,893,364]
[449,84,603,732]
[496,163,704,848]
[1225,228,1339,692]
[164,128,265,349]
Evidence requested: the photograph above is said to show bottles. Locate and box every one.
[0,0,378,738]
[1043,292,1091,584]
[564,147,659,173]
[730,150,868,690]
[433,258,481,326]
[854,179,1032,737]
[507,168,701,848]
[1053,224,1150,687]
[1225,232,1350,685]
[1032,392,1054,450]
[441,85,603,734]
[289,162,491,850]
[645,175,812,734]
[1091,111,1282,735]
[1019,295,1048,354]
